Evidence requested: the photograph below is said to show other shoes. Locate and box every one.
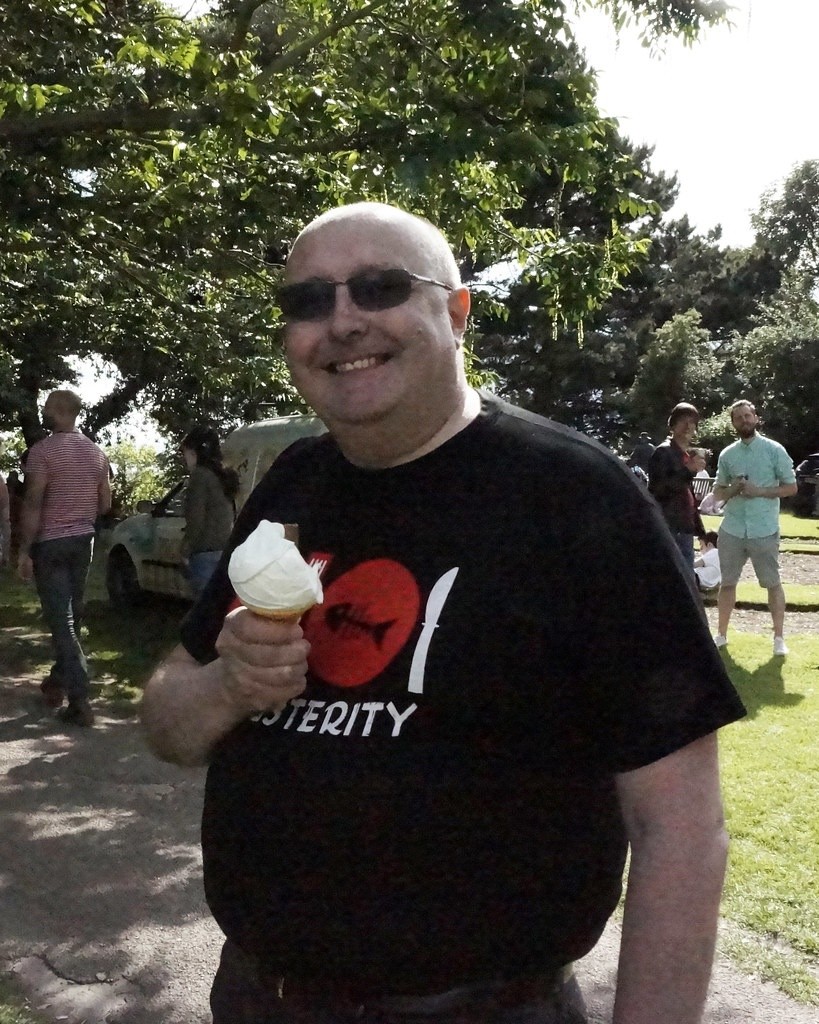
[58,703,93,727]
[773,637,787,656]
[40,679,63,706]
[714,633,728,647]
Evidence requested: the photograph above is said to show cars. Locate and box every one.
[793,453,819,517]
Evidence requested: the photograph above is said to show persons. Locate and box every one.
[0,448,31,569]
[18,391,112,727]
[179,427,240,607]
[647,401,708,581]
[712,399,798,637]
[135,199,750,1023]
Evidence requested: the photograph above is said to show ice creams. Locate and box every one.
[697,448,705,459]
[227,519,324,626]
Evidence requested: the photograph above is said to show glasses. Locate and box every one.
[273,268,452,322]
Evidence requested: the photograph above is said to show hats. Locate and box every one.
[639,432,651,440]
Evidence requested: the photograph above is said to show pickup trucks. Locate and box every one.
[106,415,327,618]
